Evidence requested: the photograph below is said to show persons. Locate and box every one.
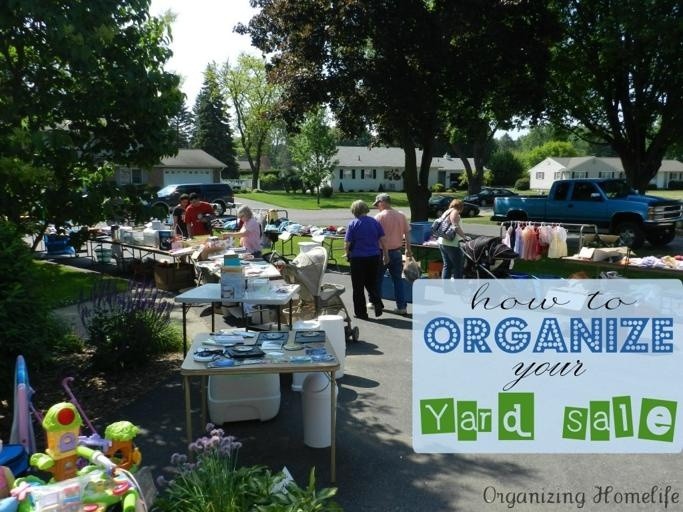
[184,192,216,240]
[438,199,467,278]
[173,193,190,238]
[342,200,389,320]
[223,206,262,258]
[366,193,412,315]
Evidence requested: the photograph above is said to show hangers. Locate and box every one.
[504,216,564,233]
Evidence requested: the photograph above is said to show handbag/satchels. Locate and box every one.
[431,210,456,241]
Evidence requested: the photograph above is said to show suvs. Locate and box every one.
[148,181,236,220]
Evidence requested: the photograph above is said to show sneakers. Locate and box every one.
[367,303,385,310]
[393,308,407,315]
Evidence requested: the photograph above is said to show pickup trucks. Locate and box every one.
[488,176,682,252]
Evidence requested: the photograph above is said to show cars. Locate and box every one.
[428,193,482,220]
[463,186,520,209]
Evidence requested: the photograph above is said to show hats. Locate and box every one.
[372,194,390,206]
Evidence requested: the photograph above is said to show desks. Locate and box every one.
[557,256,682,281]
[64,205,521,488]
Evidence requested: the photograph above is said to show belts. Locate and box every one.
[380,248,400,251]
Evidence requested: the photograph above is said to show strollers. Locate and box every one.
[456,234,520,279]
[313,282,361,346]
[28,375,103,468]
